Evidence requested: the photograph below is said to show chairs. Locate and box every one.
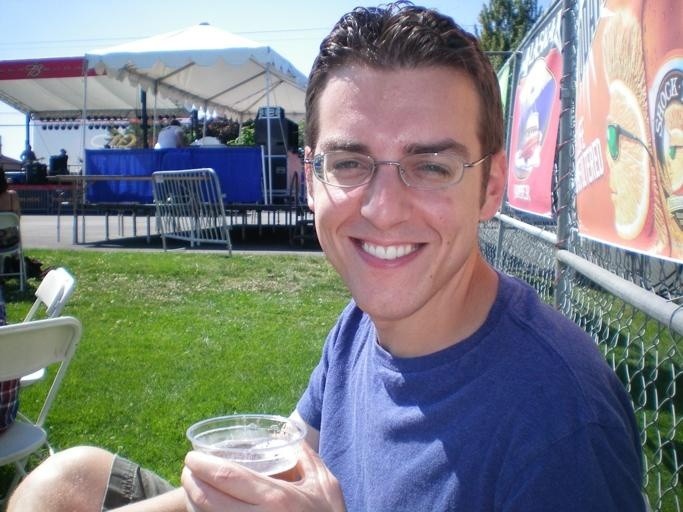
[0,210,84,512]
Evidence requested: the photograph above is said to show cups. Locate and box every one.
[186,414,307,480]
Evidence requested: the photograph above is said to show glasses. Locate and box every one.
[303,147,492,190]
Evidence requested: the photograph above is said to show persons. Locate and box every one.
[192,130,221,145]
[21,145,38,165]
[155,120,186,149]
[0,168,21,285]
[5,0,643,512]
[0,290,20,434]
